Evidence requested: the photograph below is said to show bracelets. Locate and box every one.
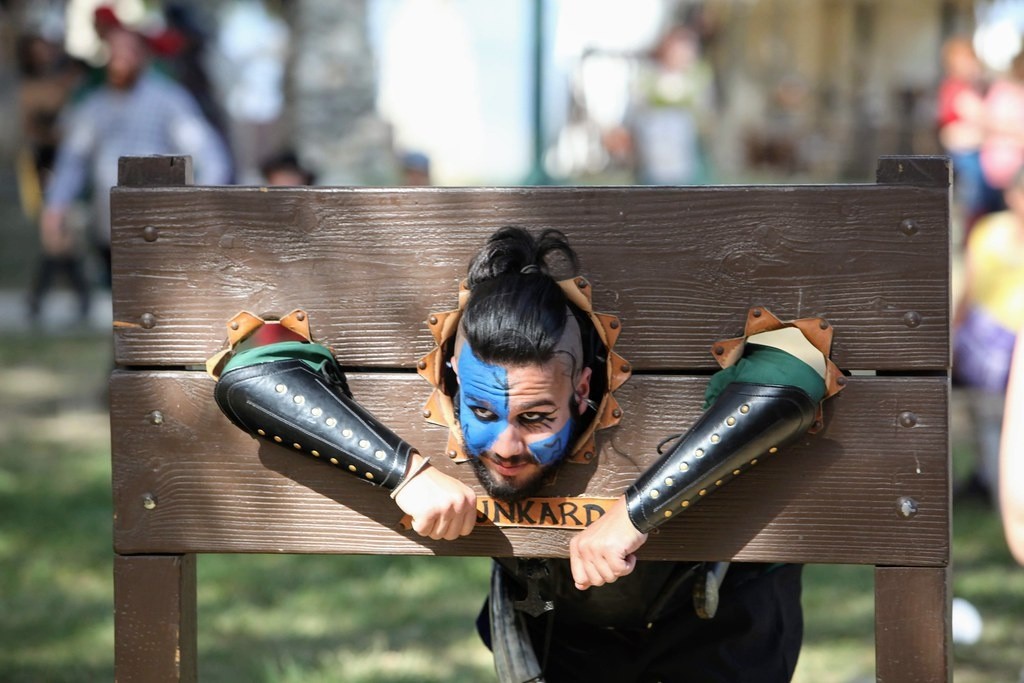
[389,456,432,500]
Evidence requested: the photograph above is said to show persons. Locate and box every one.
[936,34,1004,254]
[14,32,94,330]
[210,224,852,683]
[34,7,236,414]
[598,17,726,189]
[261,141,316,188]
[946,49,1024,510]
